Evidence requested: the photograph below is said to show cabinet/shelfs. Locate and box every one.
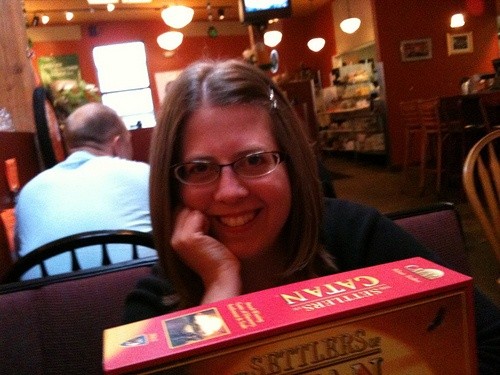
[318,41,378,116]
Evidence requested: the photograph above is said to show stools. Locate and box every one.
[399,97,465,191]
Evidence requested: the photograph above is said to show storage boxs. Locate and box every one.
[99,257,474,375]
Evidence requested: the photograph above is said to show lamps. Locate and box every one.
[340,0,360,34]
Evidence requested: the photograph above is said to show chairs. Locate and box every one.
[0,131,500,375]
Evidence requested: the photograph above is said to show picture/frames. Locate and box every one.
[401,39,432,62]
[446,32,474,55]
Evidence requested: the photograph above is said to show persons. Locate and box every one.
[14,103,157,278]
[122,58,500,375]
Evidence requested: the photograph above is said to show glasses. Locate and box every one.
[173,150,286,185]
